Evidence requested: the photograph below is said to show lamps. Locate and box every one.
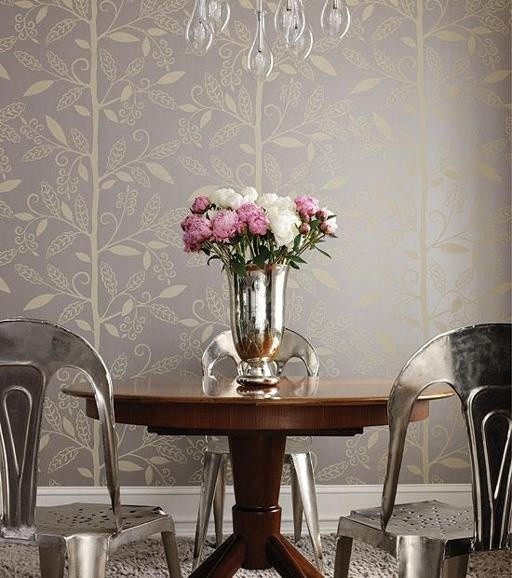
[186,0,351,80]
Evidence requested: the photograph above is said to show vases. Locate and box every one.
[225,265,291,384]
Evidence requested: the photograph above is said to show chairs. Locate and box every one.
[333,323,512,578]
[0,319,184,578]
[193,326,329,571]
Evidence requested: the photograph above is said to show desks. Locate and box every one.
[61,378,455,578]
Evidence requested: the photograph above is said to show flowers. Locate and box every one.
[182,187,339,338]
